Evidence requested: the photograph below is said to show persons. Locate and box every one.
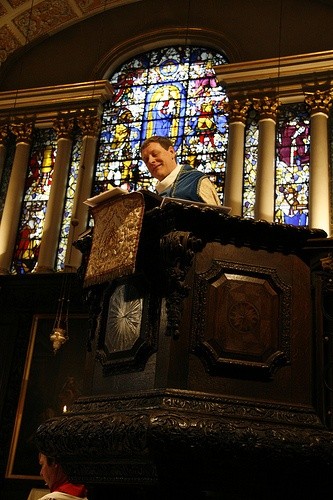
[29,417,94,500]
[141,136,222,207]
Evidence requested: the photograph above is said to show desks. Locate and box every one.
[64,188,333,436]
